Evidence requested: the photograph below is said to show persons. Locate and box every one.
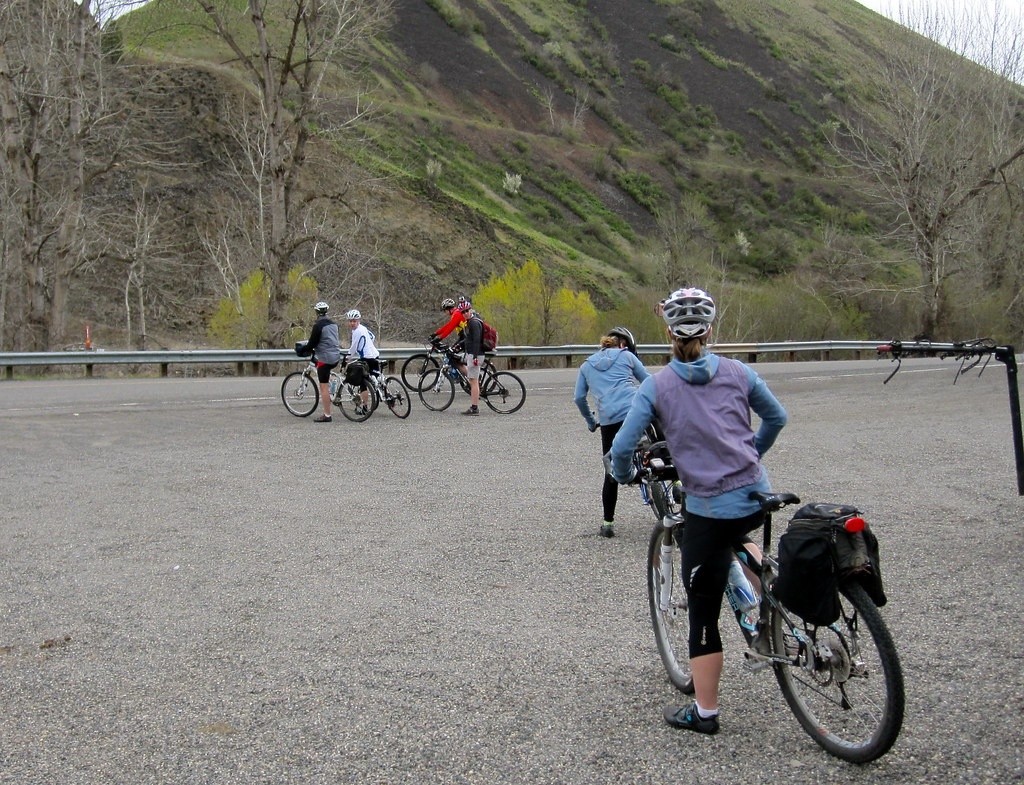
[610,287,786,735]
[296,302,340,422]
[428,298,468,392]
[456,301,485,415]
[574,327,651,538]
[346,309,379,415]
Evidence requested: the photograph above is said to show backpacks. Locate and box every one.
[473,317,498,350]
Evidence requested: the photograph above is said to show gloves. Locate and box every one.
[433,336,442,343]
[455,344,461,349]
[429,332,437,340]
[473,358,478,366]
[621,469,647,486]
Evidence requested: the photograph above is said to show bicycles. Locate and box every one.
[329,351,412,419]
[402,332,497,397]
[601,440,905,762]
[417,339,526,414]
[589,406,675,520]
[281,340,375,422]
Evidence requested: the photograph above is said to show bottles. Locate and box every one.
[722,552,759,612]
[330,379,337,394]
[371,375,381,387]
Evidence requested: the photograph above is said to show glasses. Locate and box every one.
[461,308,470,314]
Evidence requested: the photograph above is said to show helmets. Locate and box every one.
[314,301,330,311]
[608,326,636,353]
[441,298,455,310]
[662,287,716,338]
[458,296,472,303]
[458,301,472,312]
[346,309,361,319]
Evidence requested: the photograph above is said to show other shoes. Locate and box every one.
[356,410,368,415]
[479,374,482,381]
[460,407,480,416]
[313,414,332,422]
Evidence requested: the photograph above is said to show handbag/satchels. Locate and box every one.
[774,503,887,626]
[346,361,369,386]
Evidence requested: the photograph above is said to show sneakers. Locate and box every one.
[662,703,720,734]
[598,524,615,538]
